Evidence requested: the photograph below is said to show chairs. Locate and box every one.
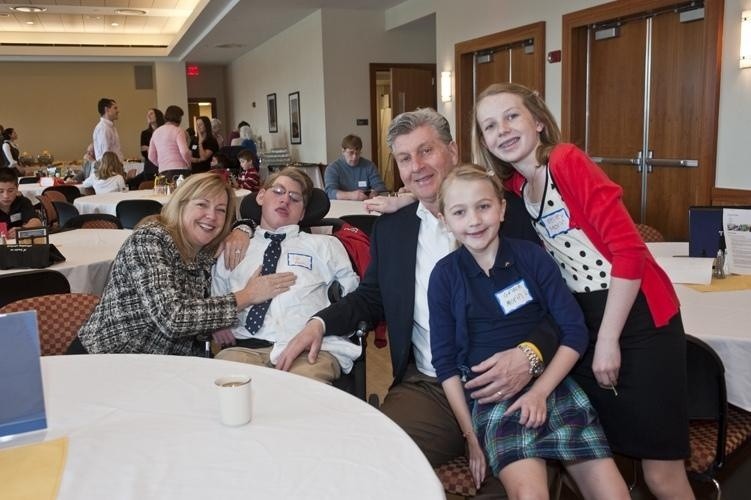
[40,185,84,204]
[669,333,751,500]
[354,306,581,499]
[31,201,82,229]
[629,223,664,241]
[115,199,163,231]
[202,219,366,403]
[138,181,154,190]
[80,219,119,231]
[1,294,102,357]
[2,270,69,307]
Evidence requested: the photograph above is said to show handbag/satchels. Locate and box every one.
[686,331,729,485]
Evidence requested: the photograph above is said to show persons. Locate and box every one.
[147,105,192,184]
[211,118,225,149]
[187,115,216,174]
[230,149,260,193]
[0,167,43,240]
[70,143,94,184]
[140,108,165,172]
[92,151,132,195]
[473,81,695,500]
[206,167,355,378]
[323,134,385,201]
[0,127,26,176]
[93,98,122,165]
[428,161,629,500]
[209,154,230,186]
[272,106,562,467]
[62,171,297,358]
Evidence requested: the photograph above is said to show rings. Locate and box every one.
[235,249,242,256]
[494,390,505,399]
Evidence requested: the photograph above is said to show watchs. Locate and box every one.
[515,342,546,378]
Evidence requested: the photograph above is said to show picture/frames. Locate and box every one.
[288,92,301,145]
[267,93,278,133]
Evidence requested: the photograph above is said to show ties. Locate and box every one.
[243,231,286,335]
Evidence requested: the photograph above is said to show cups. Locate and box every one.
[214,375,252,426]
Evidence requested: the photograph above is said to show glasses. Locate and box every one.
[267,184,303,204]
